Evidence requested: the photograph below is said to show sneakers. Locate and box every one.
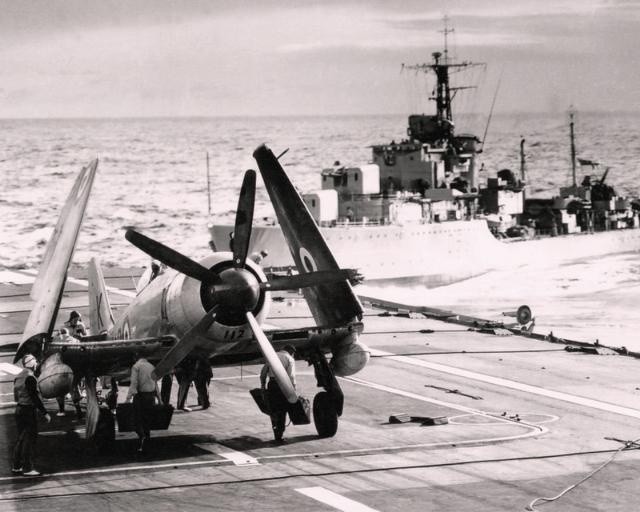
[137,437,149,451]
[11,467,40,475]
[56,412,66,417]
[75,411,82,416]
[276,437,287,441]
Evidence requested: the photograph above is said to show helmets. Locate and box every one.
[151,261,161,273]
[70,311,81,321]
[61,328,68,334]
[23,355,36,367]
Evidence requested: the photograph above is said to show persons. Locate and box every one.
[11,354,52,476]
[61,310,87,339]
[161,359,213,412]
[56,384,82,417]
[260,344,296,442]
[126,349,164,452]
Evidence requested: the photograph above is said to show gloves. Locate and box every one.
[44,414,51,423]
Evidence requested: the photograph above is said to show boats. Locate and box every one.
[209,19,640,304]
